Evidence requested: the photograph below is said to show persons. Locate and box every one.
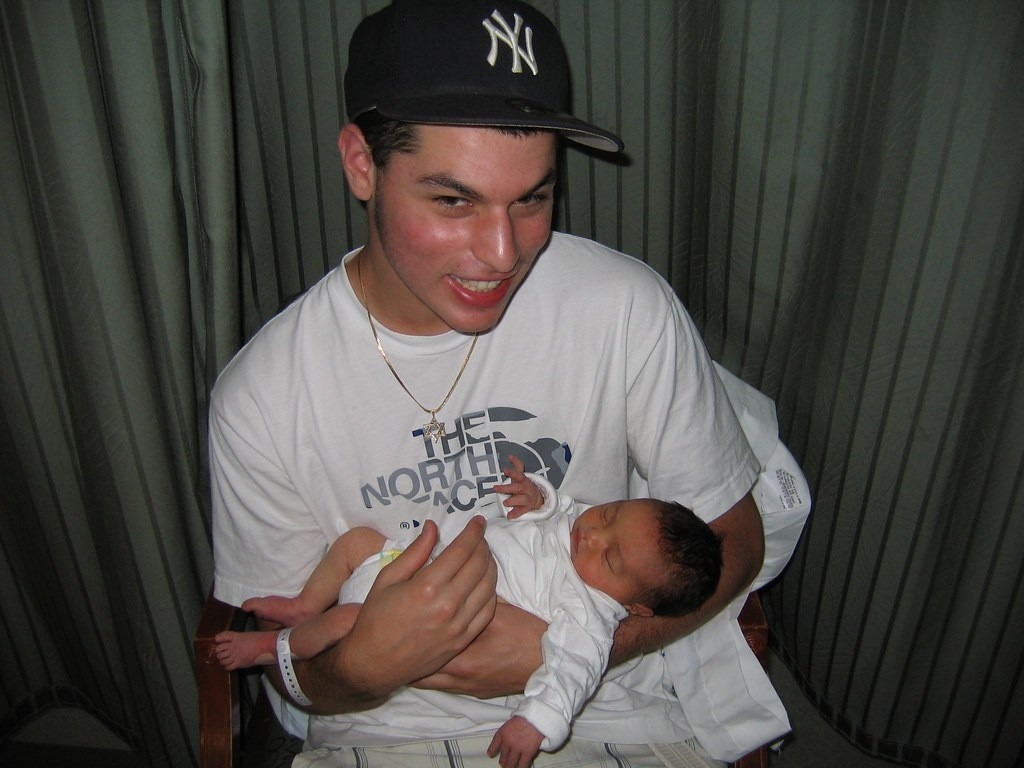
[214,452,722,768]
[208,0,766,768]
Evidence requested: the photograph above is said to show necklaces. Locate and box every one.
[355,253,478,444]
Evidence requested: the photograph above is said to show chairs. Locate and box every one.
[193,588,771,768]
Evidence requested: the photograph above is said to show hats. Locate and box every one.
[343,0,625,156]
